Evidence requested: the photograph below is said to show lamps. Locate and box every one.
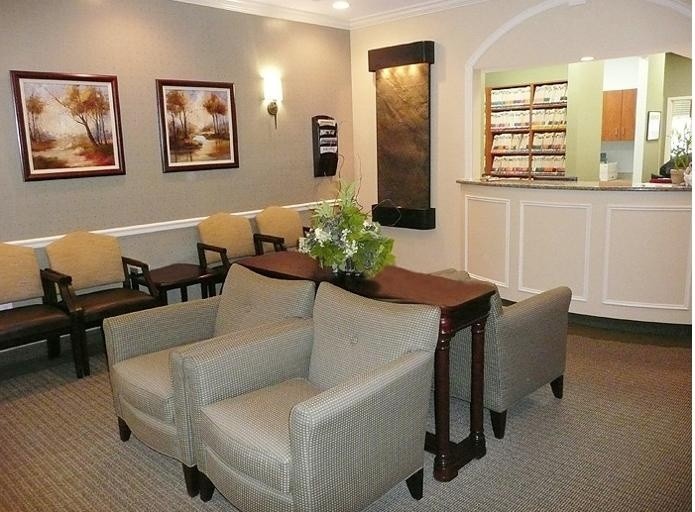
[261,62,281,131]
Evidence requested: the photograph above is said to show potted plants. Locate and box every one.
[668,122,692,183]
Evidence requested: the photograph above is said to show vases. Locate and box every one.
[328,245,366,278]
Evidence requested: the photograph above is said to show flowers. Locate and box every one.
[296,153,396,279]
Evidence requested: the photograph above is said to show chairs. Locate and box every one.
[426,268,458,278]
[254,203,310,251]
[43,229,164,377]
[0,240,85,380]
[180,279,443,511]
[431,270,574,439]
[194,211,281,298]
[103,261,317,499]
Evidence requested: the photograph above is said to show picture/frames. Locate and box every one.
[8,68,126,182]
[646,109,661,142]
[154,78,240,173]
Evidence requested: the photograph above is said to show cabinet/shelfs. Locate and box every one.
[602,87,636,142]
[485,79,568,178]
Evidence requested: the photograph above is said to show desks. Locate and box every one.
[233,249,497,480]
[137,262,218,305]
